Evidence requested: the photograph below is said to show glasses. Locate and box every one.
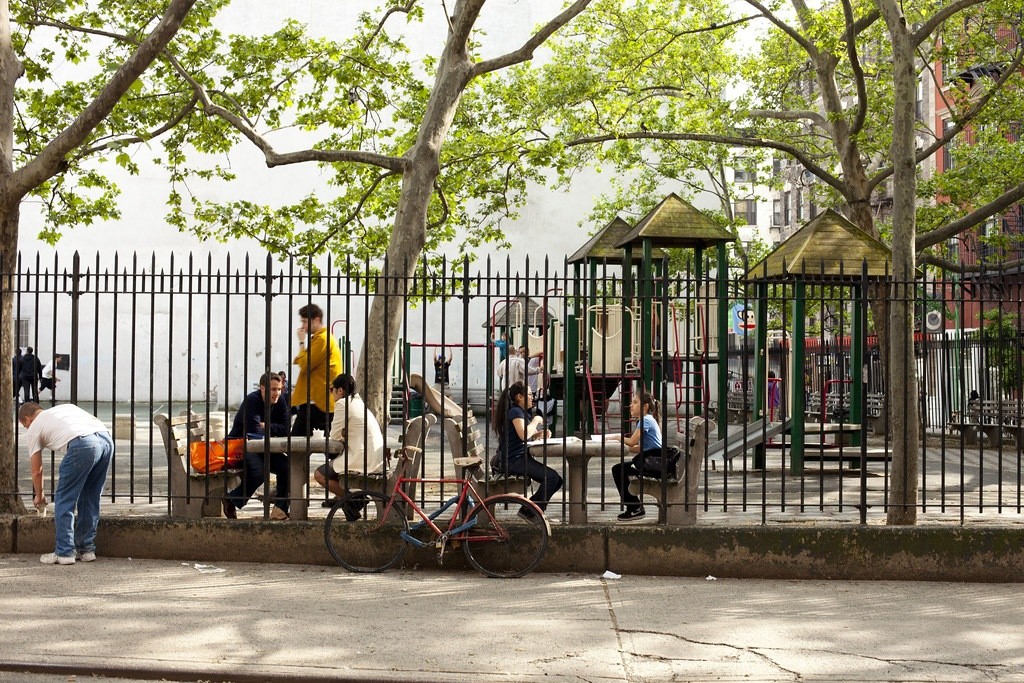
[330,387,336,392]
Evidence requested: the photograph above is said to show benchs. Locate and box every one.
[339,413,436,522]
[628,417,708,523]
[155,412,244,519]
[947,399,1024,448]
[443,415,531,522]
[804,392,886,435]
[711,393,754,423]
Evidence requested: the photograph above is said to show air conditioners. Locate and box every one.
[877,178,894,202]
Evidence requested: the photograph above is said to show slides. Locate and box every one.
[409,373,463,418]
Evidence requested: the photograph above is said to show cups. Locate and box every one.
[313,430,324,438]
[37,504,46,518]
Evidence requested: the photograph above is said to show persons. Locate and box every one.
[221,372,291,519]
[18,401,114,565]
[38,353,62,403]
[518,346,544,418]
[805,367,812,392]
[433,347,453,400]
[278,371,292,405]
[258,303,342,504]
[967,390,980,412]
[12,348,24,404]
[18,347,42,404]
[490,331,511,396]
[491,380,563,526]
[315,373,389,512]
[768,371,780,441]
[605,392,662,521]
[496,345,544,390]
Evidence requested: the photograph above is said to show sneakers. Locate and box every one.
[40,552,75,564]
[617,508,645,520]
[74,549,96,561]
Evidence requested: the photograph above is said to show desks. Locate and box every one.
[245,436,344,520]
[528,439,630,524]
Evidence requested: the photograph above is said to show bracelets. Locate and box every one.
[300,342,304,345]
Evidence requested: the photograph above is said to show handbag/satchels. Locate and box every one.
[189,438,245,474]
[643,456,668,479]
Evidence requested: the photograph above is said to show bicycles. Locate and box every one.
[323,445,551,579]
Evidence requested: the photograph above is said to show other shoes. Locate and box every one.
[221,493,237,519]
[517,505,537,525]
[354,496,370,511]
[286,512,289,518]
[49,398,57,403]
[322,497,344,508]
